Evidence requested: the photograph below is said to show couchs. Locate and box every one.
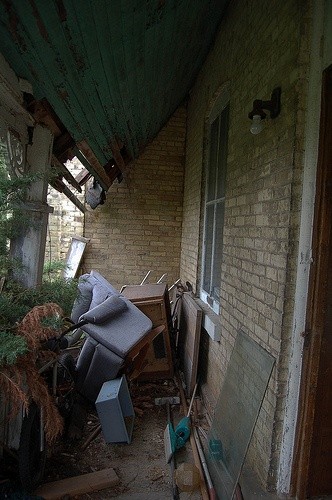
[56,268,153,410]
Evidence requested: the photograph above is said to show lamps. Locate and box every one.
[246,86,282,136]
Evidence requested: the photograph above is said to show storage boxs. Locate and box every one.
[94,375,137,444]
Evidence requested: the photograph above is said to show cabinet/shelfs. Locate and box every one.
[119,282,176,376]
